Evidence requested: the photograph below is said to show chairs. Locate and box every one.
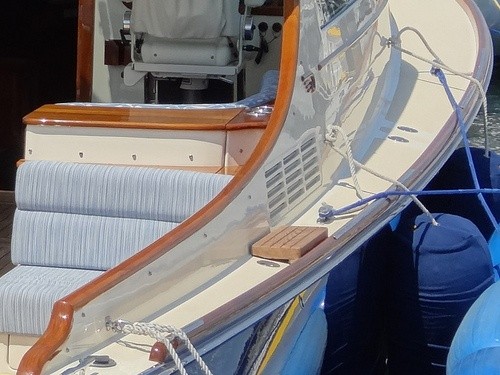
[118,0,258,103]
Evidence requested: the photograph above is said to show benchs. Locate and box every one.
[0,159,236,337]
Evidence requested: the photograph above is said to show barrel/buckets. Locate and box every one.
[308,217,372,375]
[371,202,495,375]
[423,144,499,243]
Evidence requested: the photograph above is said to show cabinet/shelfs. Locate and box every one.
[224,106,272,167]
[23,104,244,167]
